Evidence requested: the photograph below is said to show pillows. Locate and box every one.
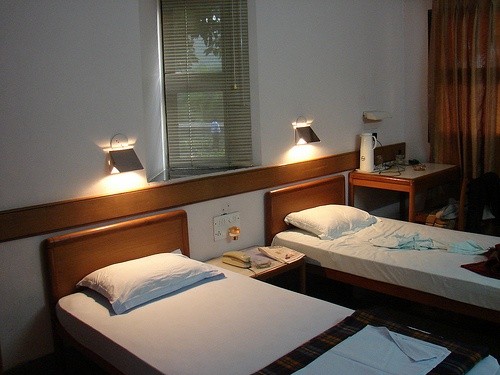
[75,249,224,313]
[284,205,376,240]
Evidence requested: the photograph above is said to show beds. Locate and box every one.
[265,174,500,326]
[48,209,500,375]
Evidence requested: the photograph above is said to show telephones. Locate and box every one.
[222,250,252,268]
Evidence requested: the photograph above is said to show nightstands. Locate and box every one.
[203,245,305,295]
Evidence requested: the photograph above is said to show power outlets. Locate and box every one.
[214,212,240,241]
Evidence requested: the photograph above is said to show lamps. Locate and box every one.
[106,132,144,175]
[294,116,321,145]
[228,226,240,241]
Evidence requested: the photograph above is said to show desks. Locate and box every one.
[348,161,457,222]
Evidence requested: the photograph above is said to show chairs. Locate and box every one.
[414,172,491,231]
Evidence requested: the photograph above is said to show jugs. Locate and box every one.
[360,133,378,172]
